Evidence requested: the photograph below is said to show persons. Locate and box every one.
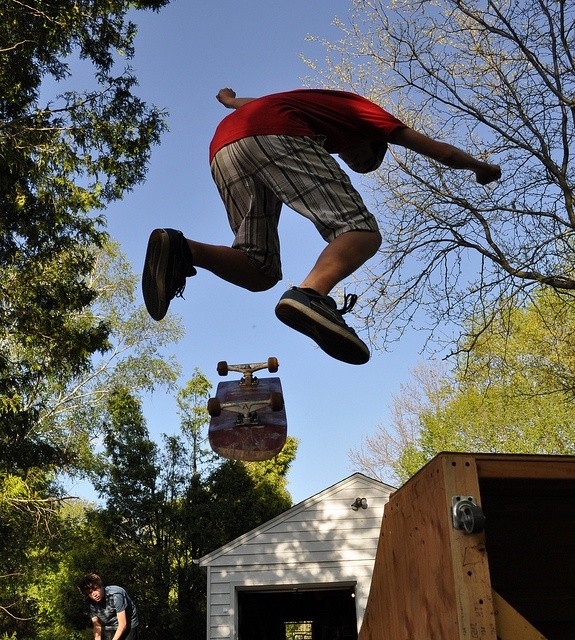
[79,573,139,640]
[142,87,501,365]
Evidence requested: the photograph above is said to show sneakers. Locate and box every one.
[142,227,197,321]
[274,286,370,365]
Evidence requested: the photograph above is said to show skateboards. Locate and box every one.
[207,357,288,462]
